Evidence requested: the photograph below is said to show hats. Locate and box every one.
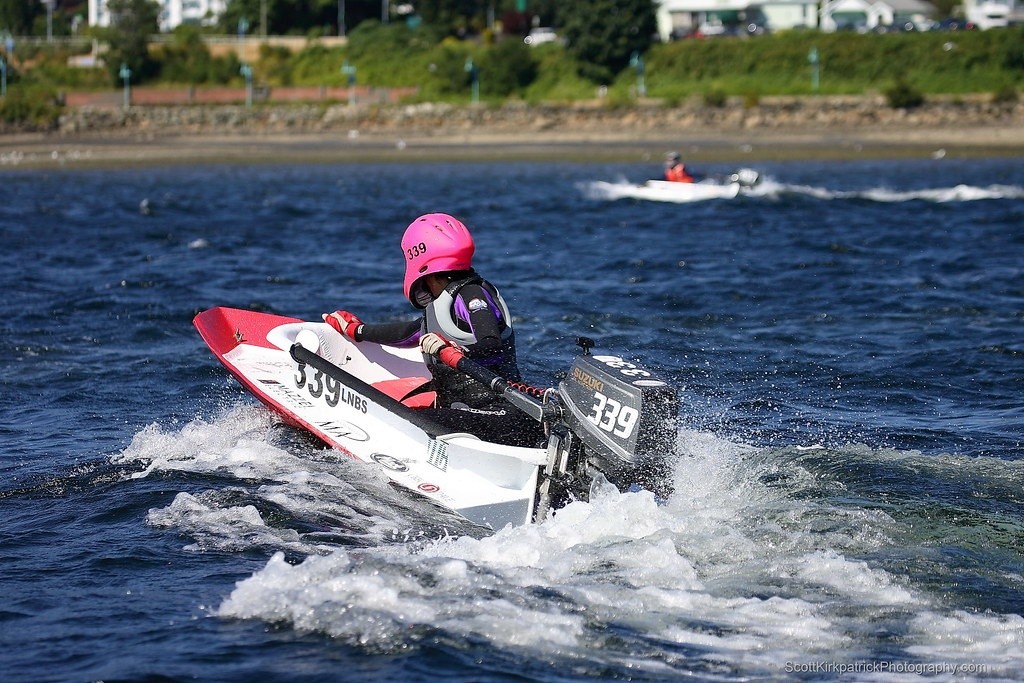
[668,152,681,161]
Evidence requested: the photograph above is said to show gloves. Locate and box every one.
[418,332,465,360]
[322,310,366,343]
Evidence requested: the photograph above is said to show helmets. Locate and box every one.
[400,212,476,310]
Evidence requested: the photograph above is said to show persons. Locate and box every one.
[321,213,541,447]
[656,150,707,183]
